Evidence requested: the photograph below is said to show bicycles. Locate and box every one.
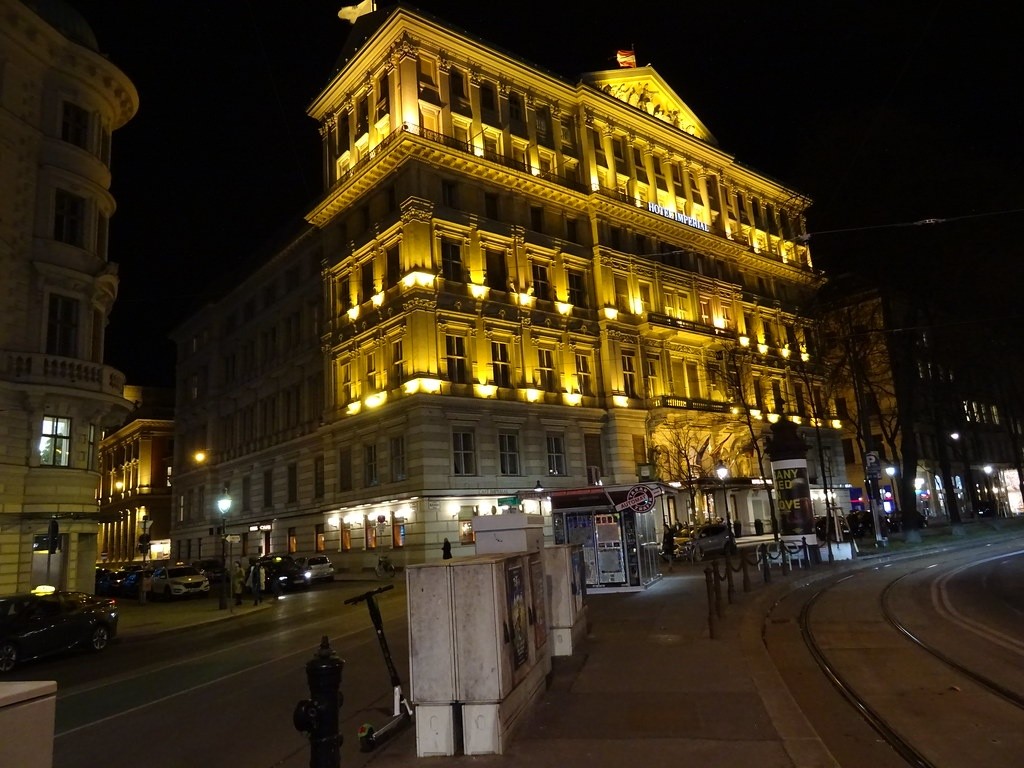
[375,556,395,578]
[676,536,705,566]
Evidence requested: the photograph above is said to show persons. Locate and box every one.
[440,537,452,560]
[846,509,872,538]
[663,525,674,573]
[674,519,688,532]
[252,560,265,607]
[231,561,245,605]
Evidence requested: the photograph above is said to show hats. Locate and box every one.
[256,560,263,563]
[234,559,241,562]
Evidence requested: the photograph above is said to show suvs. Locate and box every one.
[665,523,737,561]
[150,566,210,600]
[187,559,227,582]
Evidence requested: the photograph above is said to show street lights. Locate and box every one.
[533,480,544,515]
[217,488,232,610]
[716,464,737,555]
[885,467,899,511]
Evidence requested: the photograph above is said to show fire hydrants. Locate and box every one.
[294,636,345,768]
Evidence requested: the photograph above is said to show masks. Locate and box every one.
[255,563,261,566]
[235,563,239,567]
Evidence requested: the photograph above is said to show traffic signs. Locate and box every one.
[225,534,241,543]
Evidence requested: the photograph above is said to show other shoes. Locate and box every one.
[253,603,259,605]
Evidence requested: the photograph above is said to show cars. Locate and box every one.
[291,554,335,584]
[814,510,929,541]
[247,560,311,592]
[0,591,118,673]
[96,564,152,595]
